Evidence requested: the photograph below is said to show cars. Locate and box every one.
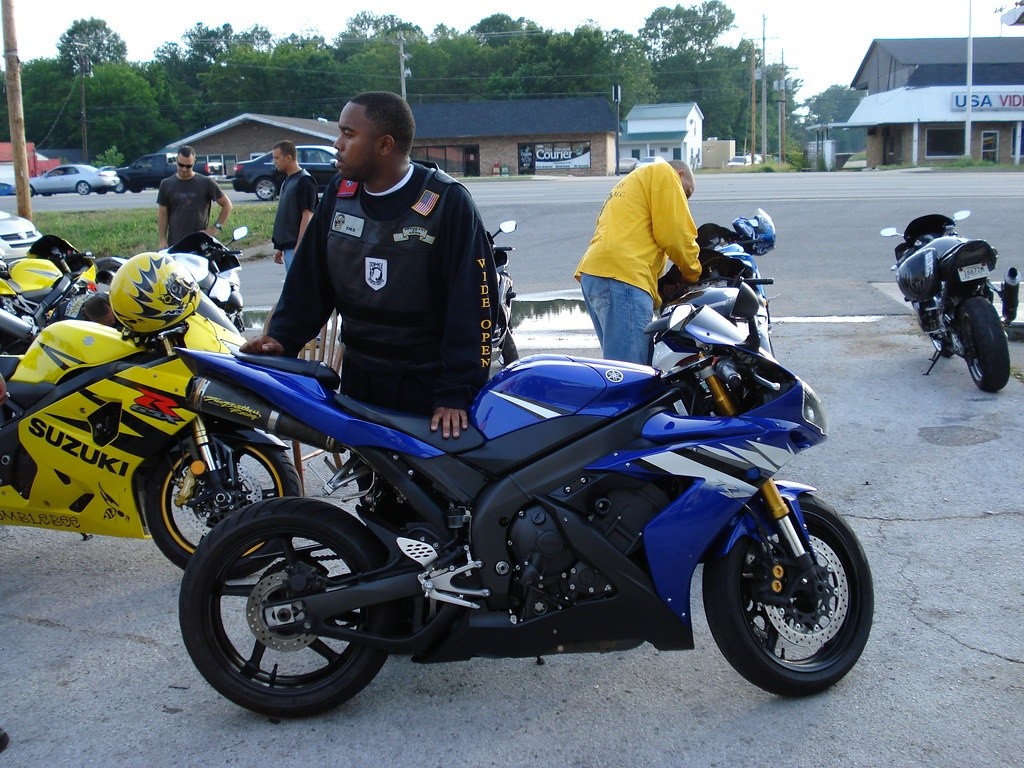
[29,164,120,197]
[636,156,666,170]
[232,144,342,202]
[0,210,42,276]
[615,158,638,174]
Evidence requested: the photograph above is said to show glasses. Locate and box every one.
[177,161,194,168]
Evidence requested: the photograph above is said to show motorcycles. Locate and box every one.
[652,224,777,372]
[171,282,875,720]
[880,209,1020,393]
[0,228,302,578]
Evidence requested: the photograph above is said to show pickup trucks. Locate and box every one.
[110,152,227,194]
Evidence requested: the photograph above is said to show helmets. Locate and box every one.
[896,248,934,303]
[109,252,202,334]
[732,208,775,256]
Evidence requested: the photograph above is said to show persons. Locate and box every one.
[272,140,321,349]
[240,90,499,515]
[574,159,702,366]
[156,146,233,251]
[44,293,124,331]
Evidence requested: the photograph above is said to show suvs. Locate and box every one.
[728,154,762,168]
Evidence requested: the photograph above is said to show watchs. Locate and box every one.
[213,223,221,230]
